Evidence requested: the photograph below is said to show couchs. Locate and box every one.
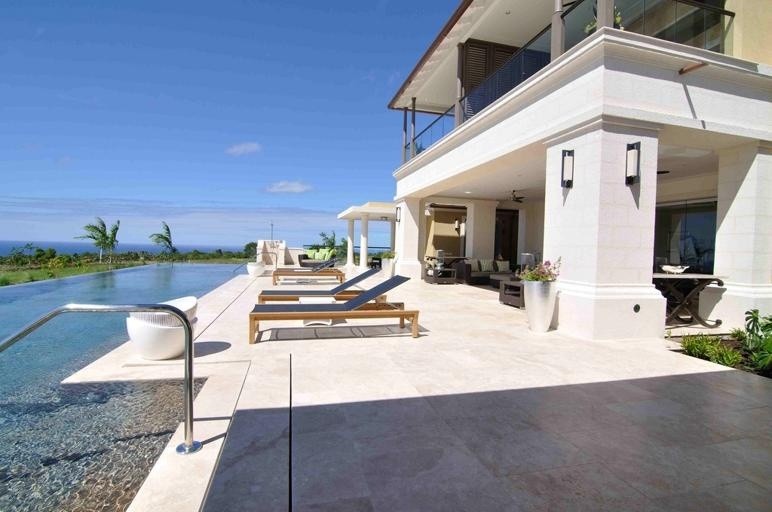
[425,254,542,308]
[298,248,337,267]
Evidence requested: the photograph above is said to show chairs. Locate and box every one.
[249,257,420,344]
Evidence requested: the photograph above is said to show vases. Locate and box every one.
[523,281,558,333]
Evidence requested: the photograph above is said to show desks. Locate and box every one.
[653,273,723,329]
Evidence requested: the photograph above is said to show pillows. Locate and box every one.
[306,247,336,260]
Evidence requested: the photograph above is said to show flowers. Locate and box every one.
[514,257,562,282]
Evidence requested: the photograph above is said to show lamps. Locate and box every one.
[626,143,640,185]
[561,149,574,189]
[396,207,401,222]
